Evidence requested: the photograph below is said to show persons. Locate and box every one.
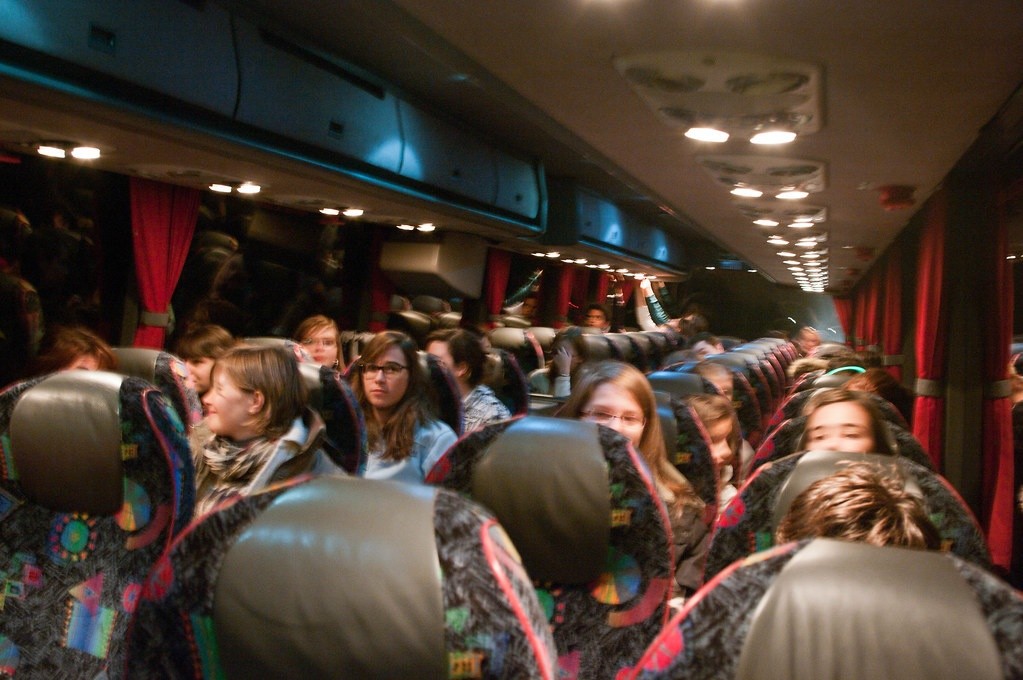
[0,152,372,385]
[27,312,511,527]
[581,270,714,356]
[771,321,939,557]
[551,334,754,608]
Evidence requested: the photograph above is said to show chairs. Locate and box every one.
[0,328,1023,680]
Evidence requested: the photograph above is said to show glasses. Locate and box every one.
[300,338,337,348]
[361,362,408,375]
[579,408,646,430]
